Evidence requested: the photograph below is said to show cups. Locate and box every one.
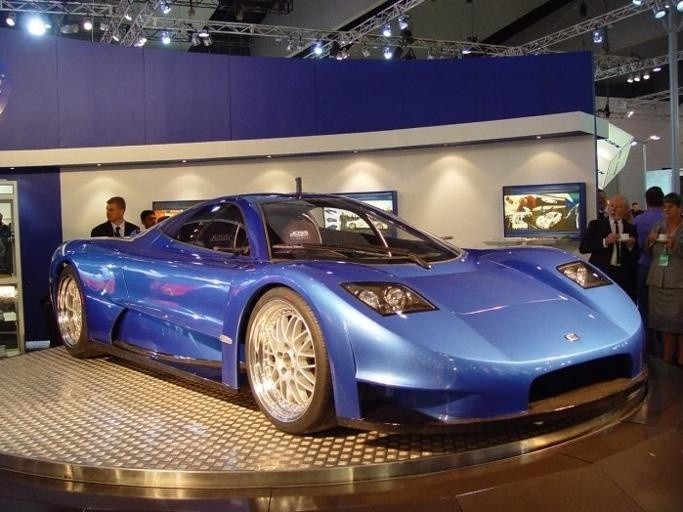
[658,234,668,239]
[620,233,629,240]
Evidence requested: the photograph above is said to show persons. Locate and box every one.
[140,210,157,229]
[579,186,683,366]
[91,197,138,237]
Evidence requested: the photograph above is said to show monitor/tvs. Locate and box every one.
[320,189,398,242]
[502,181,585,244]
[151,199,205,225]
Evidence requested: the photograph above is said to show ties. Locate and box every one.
[115,226,122,237]
[613,220,621,265]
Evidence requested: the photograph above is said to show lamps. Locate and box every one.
[591,1,682,86]
[5,0,212,51]
[275,11,473,64]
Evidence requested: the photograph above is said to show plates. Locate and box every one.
[617,239,630,242]
[654,239,668,242]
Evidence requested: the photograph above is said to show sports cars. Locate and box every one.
[48,192,654,437]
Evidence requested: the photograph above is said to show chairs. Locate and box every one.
[265,211,351,261]
[201,220,233,251]
